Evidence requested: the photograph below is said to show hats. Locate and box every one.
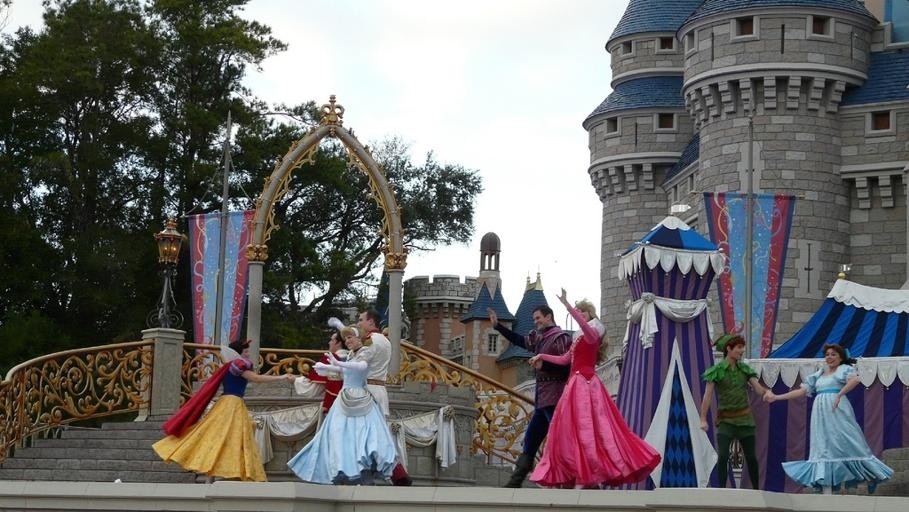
[712,333,740,351]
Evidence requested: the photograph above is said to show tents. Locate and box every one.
[714,273,909,495]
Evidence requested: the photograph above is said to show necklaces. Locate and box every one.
[352,348,361,353]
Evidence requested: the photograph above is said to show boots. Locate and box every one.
[393,465,413,486]
[503,455,532,487]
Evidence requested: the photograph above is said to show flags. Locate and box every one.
[702,190,795,360]
[186,209,255,380]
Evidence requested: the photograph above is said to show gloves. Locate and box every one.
[315,363,341,377]
[328,317,343,329]
[324,350,366,371]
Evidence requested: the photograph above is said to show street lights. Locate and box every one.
[140,214,189,331]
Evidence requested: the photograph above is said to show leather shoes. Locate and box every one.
[867,482,876,493]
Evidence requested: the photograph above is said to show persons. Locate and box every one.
[286,332,343,418]
[483,304,573,489]
[193,338,291,483]
[699,334,774,489]
[762,343,877,497]
[526,289,611,490]
[311,324,412,486]
[328,309,393,423]
